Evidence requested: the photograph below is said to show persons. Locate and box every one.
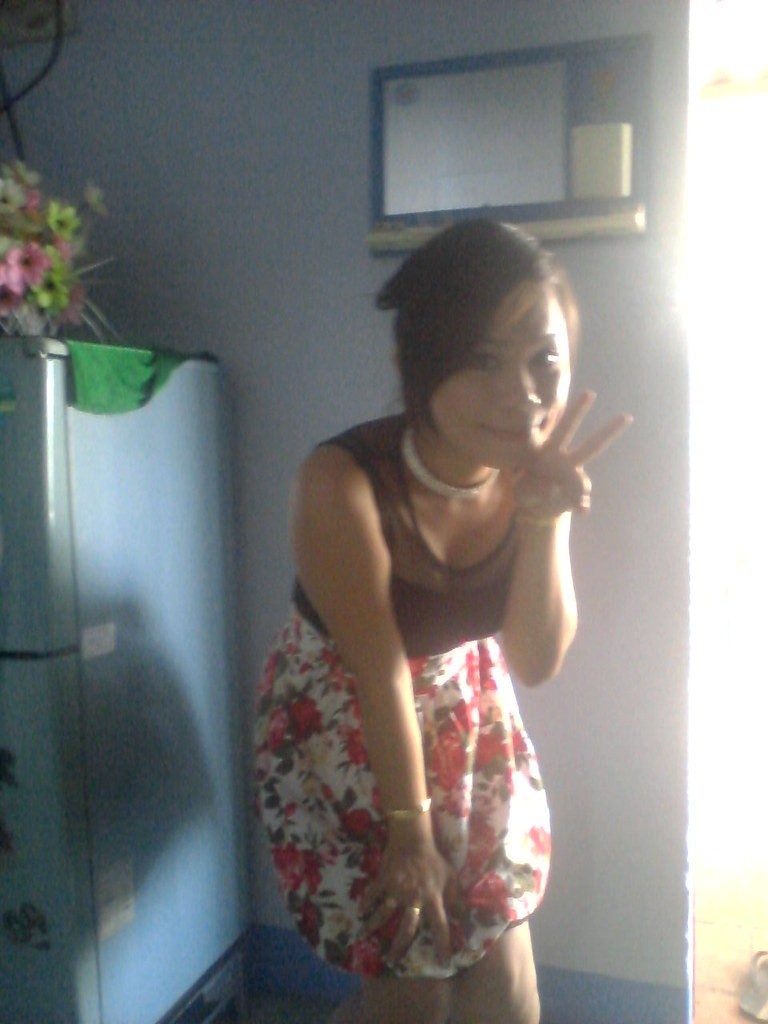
[249,218,632,1024]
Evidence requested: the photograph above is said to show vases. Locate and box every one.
[0,298,59,338]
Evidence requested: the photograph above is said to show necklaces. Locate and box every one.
[399,424,501,498]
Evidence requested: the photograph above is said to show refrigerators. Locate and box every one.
[0,334,253,1024]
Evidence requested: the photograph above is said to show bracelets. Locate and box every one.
[380,795,432,819]
[516,509,560,529]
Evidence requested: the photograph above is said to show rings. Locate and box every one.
[405,905,420,915]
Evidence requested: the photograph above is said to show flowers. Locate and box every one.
[0,161,119,342]
